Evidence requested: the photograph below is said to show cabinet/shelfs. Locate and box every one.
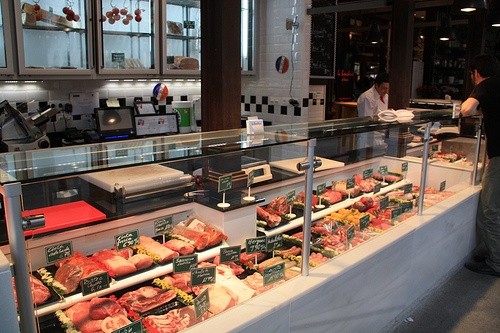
[0,0,255,80]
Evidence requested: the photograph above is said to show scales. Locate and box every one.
[242,155,273,187]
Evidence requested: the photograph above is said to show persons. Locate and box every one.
[350,71,390,147]
[460,53,500,275]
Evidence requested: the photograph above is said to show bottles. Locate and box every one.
[171,101,192,133]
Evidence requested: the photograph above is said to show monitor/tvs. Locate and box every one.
[94,106,135,134]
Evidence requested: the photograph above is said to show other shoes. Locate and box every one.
[464,262,500,277]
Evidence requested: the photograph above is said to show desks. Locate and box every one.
[335,102,357,118]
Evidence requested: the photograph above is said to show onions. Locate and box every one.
[64,7,79,21]
[103,8,142,25]
[34,3,40,10]
